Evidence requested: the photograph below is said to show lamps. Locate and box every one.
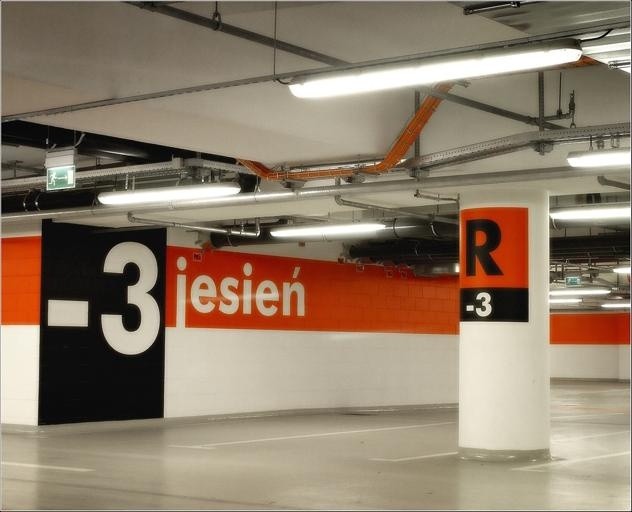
[288,31,585,101]
[563,131,630,174]
[98,174,242,208]
